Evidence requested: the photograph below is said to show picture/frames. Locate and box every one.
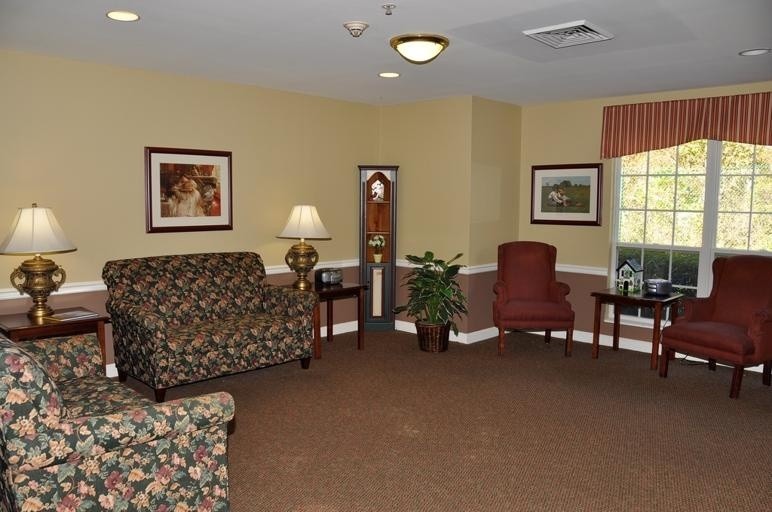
[528,162,602,226]
[142,146,234,234]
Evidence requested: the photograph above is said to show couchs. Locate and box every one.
[100,250,320,403]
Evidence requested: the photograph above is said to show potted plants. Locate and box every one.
[391,250,471,353]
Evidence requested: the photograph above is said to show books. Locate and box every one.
[41,310,100,323]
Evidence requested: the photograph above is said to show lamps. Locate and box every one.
[0,202,79,317]
[389,34,450,64]
[275,202,333,290]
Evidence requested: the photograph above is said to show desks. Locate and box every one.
[280,281,369,360]
[0,306,112,375]
[590,287,683,370]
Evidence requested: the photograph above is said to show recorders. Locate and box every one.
[314,268,343,287]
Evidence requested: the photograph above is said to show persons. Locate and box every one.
[548,183,568,206]
[372,182,384,200]
[558,185,583,206]
[167,172,207,216]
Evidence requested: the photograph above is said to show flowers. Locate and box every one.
[368,235,385,253]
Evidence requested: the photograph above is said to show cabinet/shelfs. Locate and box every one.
[356,163,400,330]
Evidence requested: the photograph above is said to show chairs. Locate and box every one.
[490,241,576,358]
[658,253,770,398]
[0,331,236,512]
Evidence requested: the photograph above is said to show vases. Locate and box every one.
[373,254,383,264]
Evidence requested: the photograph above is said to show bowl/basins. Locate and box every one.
[643,278,672,296]
